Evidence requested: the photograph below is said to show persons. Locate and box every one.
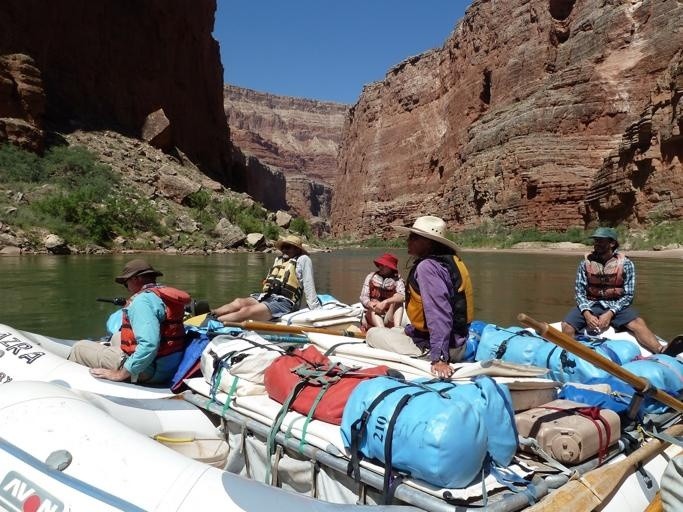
[560,227,683,359]
[365,213,475,379]
[68,259,191,384]
[211,235,320,323]
[360,254,406,327]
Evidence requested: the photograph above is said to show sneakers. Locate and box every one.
[662,335,683,357]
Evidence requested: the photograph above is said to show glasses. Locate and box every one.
[123,279,128,288]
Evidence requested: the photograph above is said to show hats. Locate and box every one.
[275,235,310,255]
[114,259,163,285]
[390,216,461,253]
[374,253,400,273]
[587,227,619,241]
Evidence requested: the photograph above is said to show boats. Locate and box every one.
[0,305,683,511]
